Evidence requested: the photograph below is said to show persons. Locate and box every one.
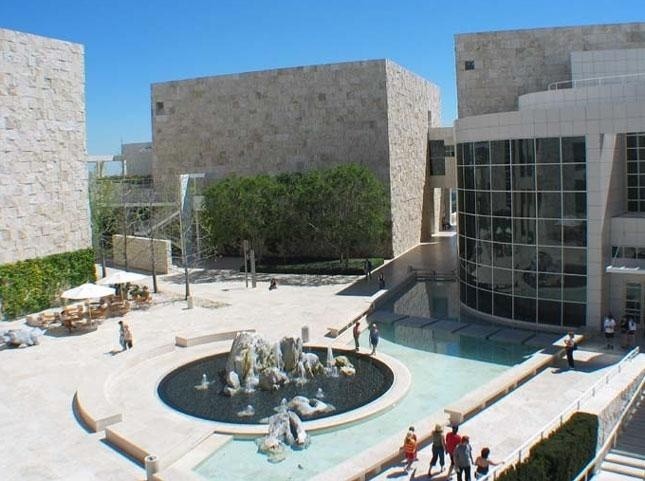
[427,424,447,477]
[364,256,372,280]
[353,321,360,352]
[37,281,150,334]
[118,321,127,351]
[602,311,637,350]
[123,325,132,348]
[445,424,462,481]
[452,434,474,481]
[398,427,420,475]
[269,277,277,290]
[563,331,575,371]
[369,322,380,356]
[472,447,505,481]
[378,271,385,289]
[442,216,452,230]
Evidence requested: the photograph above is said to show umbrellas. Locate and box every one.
[95,271,150,298]
